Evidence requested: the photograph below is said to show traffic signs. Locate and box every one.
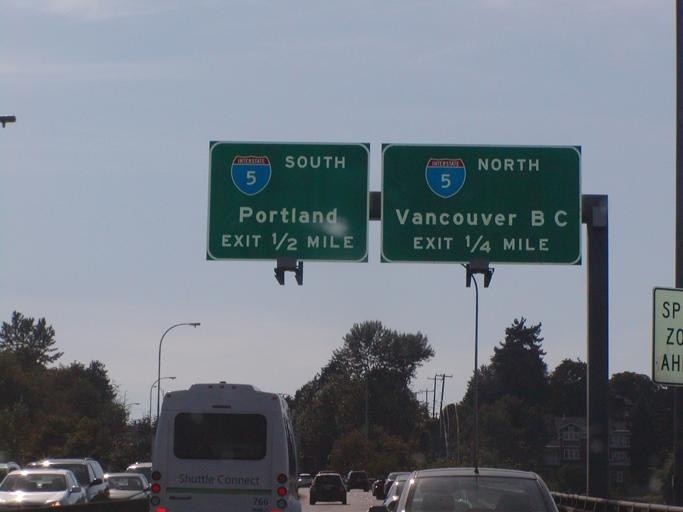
[205,140,370,263]
[380,143,583,266]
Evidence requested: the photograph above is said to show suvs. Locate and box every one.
[308,470,349,505]
[344,470,370,493]
[23,457,110,504]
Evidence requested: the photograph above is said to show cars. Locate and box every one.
[0,461,22,483]
[367,471,413,512]
[0,469,89,512]
[296,473,313,488]
[369,466,560,511]
[126,462,152,490]
[103,472,150,503]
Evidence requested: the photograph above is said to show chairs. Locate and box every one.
[419,494,454,512]
[15,478,64,490]
[128,480,138,490]
[494,494,532,512]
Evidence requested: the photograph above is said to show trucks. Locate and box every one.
[149,382,301,511]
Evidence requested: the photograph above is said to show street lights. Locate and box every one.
[0,115,17,128]
[120,403,141,423]
[156,322,201,430]
[147,376,177,426]
